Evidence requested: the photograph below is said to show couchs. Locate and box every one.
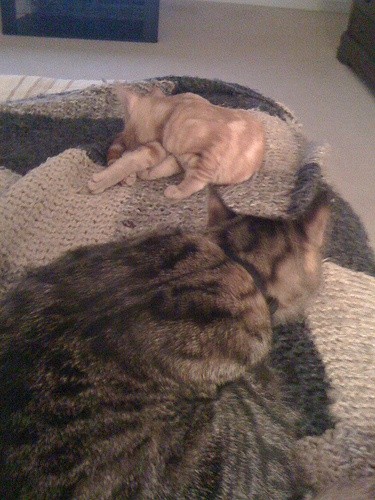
[0,75,375,499]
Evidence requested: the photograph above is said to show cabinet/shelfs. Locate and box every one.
[1,1,159,42]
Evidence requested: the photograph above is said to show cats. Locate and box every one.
[88,83,265,198]
[0,184,329,500]
